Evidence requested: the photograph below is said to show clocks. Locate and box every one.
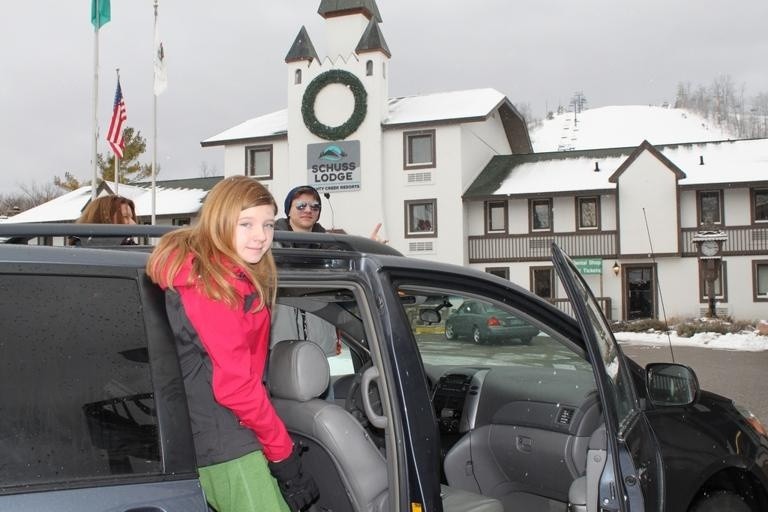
[700,240,720,257]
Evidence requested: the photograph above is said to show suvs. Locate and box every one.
[0,222,767,511]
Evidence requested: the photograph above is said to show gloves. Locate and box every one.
[266,445,320,512]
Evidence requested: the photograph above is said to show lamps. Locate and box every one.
[612,260,621,275]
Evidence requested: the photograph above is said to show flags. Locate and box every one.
[105,81,127,158]
[153,15,166,97]
[90,0,111,31]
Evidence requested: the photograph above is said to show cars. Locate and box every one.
[444,300,540,346]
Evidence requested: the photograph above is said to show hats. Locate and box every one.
[284,185,322,222]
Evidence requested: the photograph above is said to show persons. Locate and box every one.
[272,185,389,268]
[75,196,137,243]
[144,172,319,512]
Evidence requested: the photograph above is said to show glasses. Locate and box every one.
[290,200,321,210]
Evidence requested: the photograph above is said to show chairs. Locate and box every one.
[259,336,504,512]
